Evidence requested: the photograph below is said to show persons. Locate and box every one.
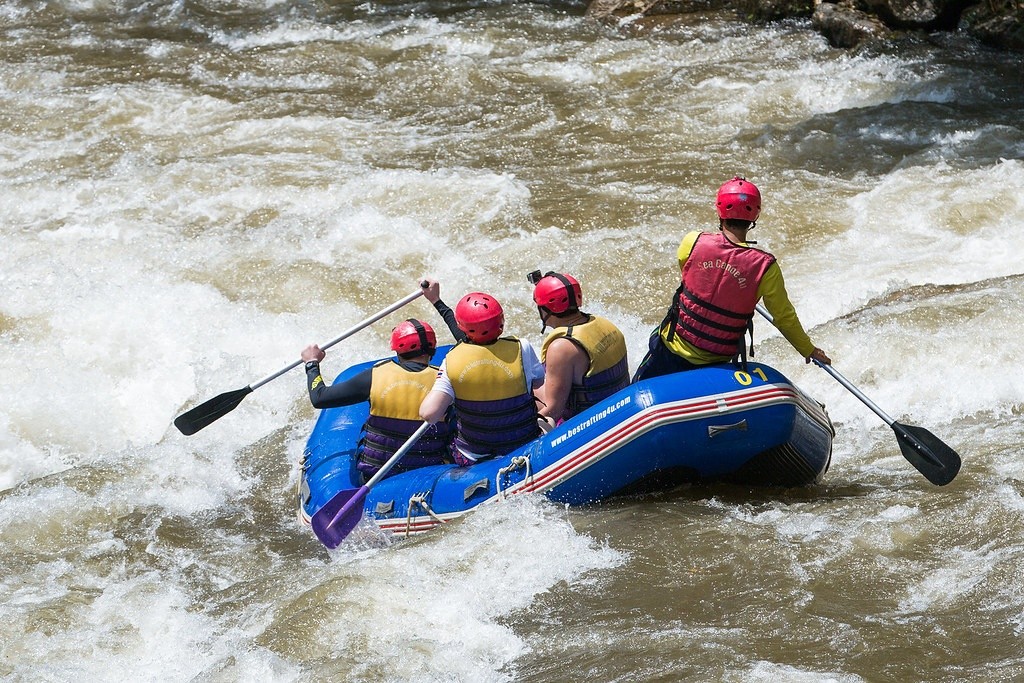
[301,269,631,485]
[632,175,831,383]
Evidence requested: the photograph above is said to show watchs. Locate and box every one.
[305,361,319,373]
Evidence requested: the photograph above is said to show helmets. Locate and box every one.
[454,291,504,344]
[716,178,761,222]
[390,318,436,360]
[533,273,582,318]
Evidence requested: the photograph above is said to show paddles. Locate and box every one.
[756,303,962,487]
[311,421,431,549]
[173,280,430,437]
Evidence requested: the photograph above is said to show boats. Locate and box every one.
[295,343,838,564]
